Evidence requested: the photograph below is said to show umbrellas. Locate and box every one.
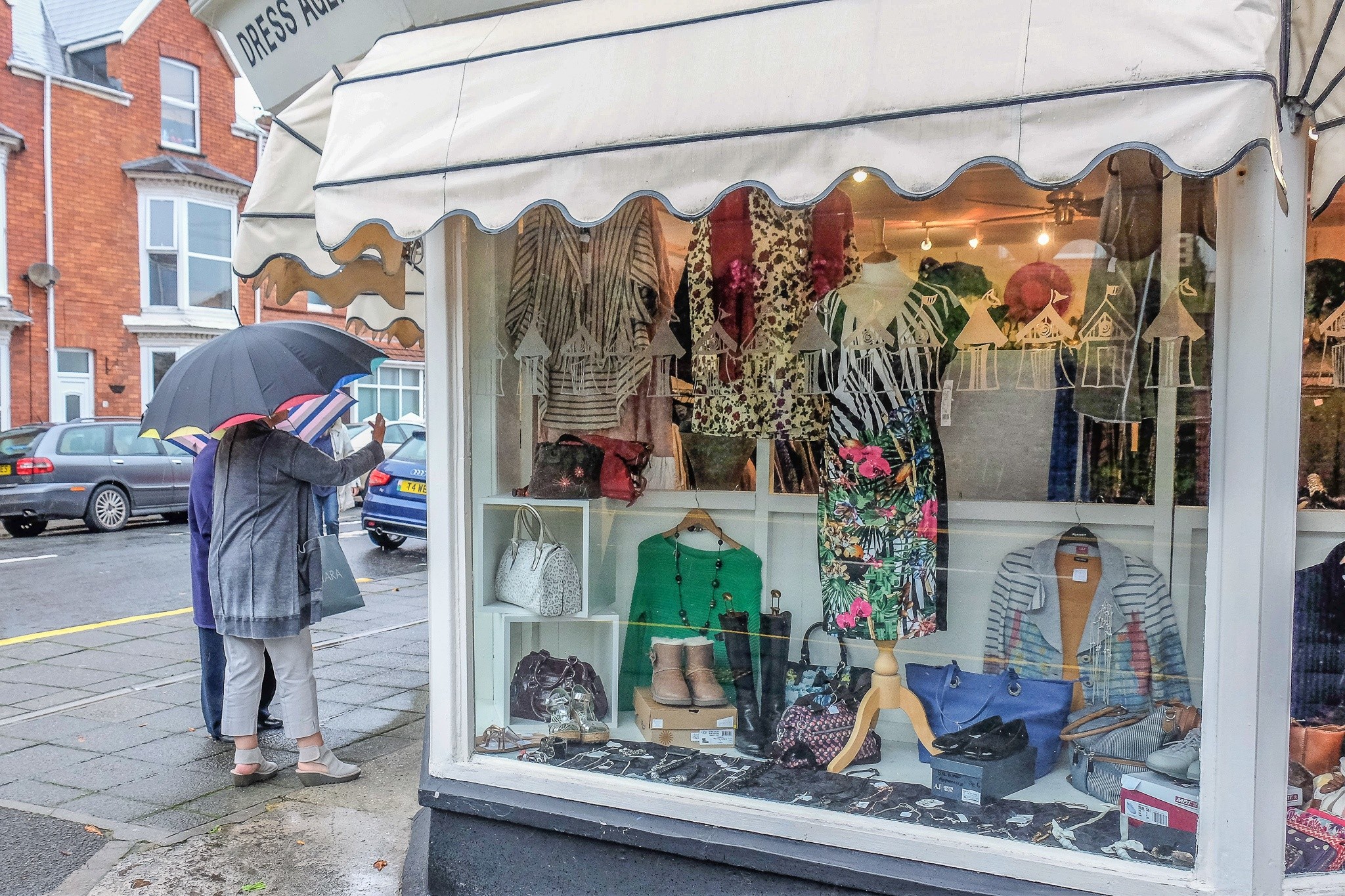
[139,306,388,461]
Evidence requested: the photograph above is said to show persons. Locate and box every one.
[808,253,971,640]
[187,406,384,786]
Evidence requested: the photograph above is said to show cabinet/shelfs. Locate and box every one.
[478,489,617,621]
[492,612,618,731]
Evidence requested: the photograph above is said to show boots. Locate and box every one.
[682,636,727,706]
[649,635,694,706]
[759,610,792,743]
[718,610,766,754]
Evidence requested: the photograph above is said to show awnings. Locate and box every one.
[227,0,1345,349]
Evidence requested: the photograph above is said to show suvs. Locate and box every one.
[0,415,196,538]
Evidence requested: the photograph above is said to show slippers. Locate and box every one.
[475,724,548,754]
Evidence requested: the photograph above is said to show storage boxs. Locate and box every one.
[1121,772,1304,839]
[633,687,739,751]
[930,744,1038,808]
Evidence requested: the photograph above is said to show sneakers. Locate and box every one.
[1146,726,1200,783]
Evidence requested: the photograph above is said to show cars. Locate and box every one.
[345,420,426,500]
[359,429,427,551]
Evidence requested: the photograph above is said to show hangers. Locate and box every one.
[1057,500,1098,551]
[662,488,740,552]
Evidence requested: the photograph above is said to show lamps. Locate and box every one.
[1037,222,1051,246]
[969,224,984,249]
[1055,210,1074,225]
[921,226,934,251]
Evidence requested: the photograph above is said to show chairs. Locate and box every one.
[71,437,88,449]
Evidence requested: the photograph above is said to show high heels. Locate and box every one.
[568,682,611,744]
[232,747,279,787]
[545,686,581,744]
[295,744,361,787]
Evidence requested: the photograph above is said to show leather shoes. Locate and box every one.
[264,713,283,729]
[212,734,234,742]
[963,718,1027,760]
[933,715,1004,752]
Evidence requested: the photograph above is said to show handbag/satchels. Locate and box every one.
[905,662,1076,779]
[1057,698,1200,806]
[317,531,366,622]
[783,621,877,709]
[525,431,651,507]
[1285,756,1345,875]
[494,503,582,617]
[1289,714,1345,780]
[769,703,882,769]
[509,648,608,723]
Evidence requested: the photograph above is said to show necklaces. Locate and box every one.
[673,524,724,633]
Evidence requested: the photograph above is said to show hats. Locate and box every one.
[1003,261,1072,322]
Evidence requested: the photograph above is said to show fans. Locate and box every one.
[964,182,1105,223]
